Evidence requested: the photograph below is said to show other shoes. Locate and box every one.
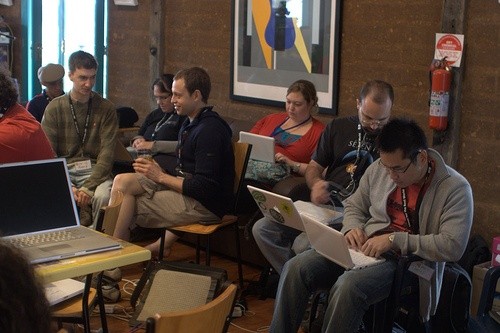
[300,289,327,332]
[80,267,123,303]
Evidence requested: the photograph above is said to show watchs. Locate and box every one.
[388,233,394,242]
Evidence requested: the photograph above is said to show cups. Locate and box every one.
[138,150,153,164]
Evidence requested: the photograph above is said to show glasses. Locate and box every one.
[378,150,420,174]
[154,92,172,100]
[358,96,392,126]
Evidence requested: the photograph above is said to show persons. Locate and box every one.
[252,80,394,275]
[144,80,330,257]
[268,115,474,333]
[0,62,56,164]
[84,68,236,303]
[25,64,65,123]
[0,243,56,333]
[125,73,188,172]
[43,51,118,229]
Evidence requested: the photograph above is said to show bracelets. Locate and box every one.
[294,163,300,173]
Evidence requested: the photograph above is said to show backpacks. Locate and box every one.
[402,261,473,333]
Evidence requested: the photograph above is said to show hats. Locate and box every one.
[37,63,65,83]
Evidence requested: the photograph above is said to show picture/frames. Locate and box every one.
[230,0,342,116]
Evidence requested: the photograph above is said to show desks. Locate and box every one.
[33,225,151,333]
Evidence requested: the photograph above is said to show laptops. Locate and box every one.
[112,141,158,163]
[245,185,342,231]
[300,213,387,271]
[41,277,86,306]
[0,158,121,267]
[240,131,278,163]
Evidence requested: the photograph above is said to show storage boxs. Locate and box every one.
[470,262,500,317]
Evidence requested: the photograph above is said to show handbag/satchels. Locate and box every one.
[257,265,279,298]
[243,158,292,186]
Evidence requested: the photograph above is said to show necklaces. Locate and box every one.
[152,113,174,136]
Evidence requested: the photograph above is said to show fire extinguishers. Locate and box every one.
[428,56,456,131]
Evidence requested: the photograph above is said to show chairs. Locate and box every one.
[48,128,500,333]
